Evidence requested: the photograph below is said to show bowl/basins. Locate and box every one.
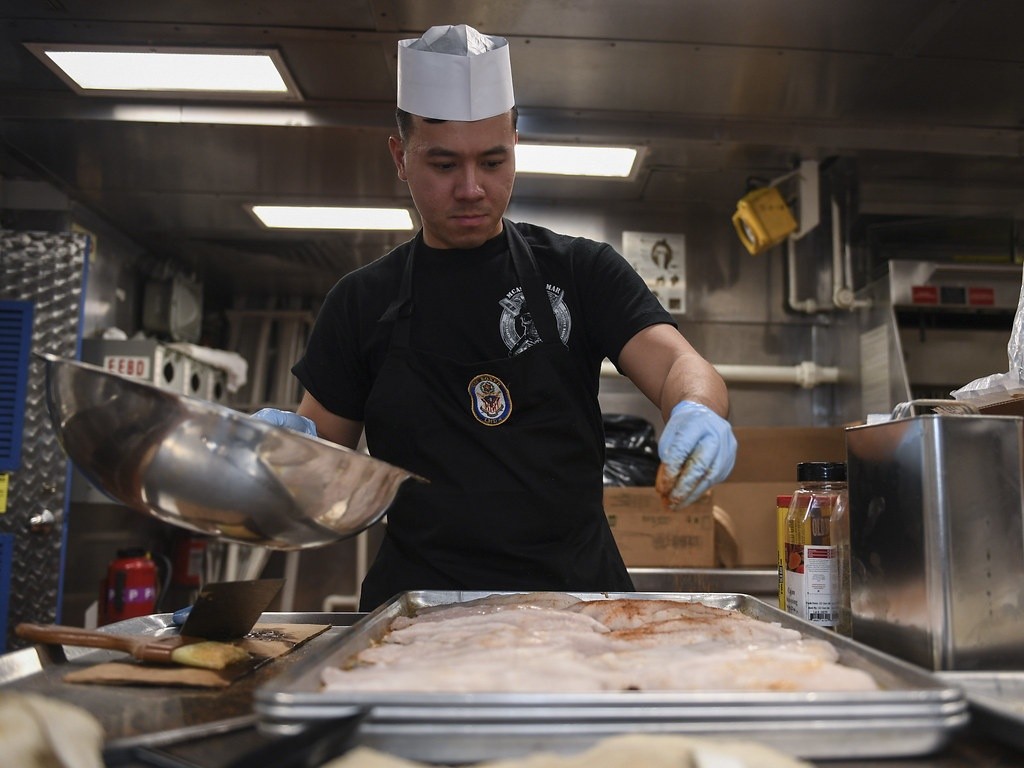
[35,352,430,553]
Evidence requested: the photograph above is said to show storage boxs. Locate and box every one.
[716,481,800,569]
[602,485,717,568]
[724,426,863,484]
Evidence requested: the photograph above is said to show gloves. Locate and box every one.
[655,401,740,510]
[251,408,317,439]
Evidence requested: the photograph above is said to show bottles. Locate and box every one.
[785,462,852,637]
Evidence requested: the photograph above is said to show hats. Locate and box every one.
[395,24,517,123]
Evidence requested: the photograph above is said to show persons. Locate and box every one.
[250,22,739,624]
